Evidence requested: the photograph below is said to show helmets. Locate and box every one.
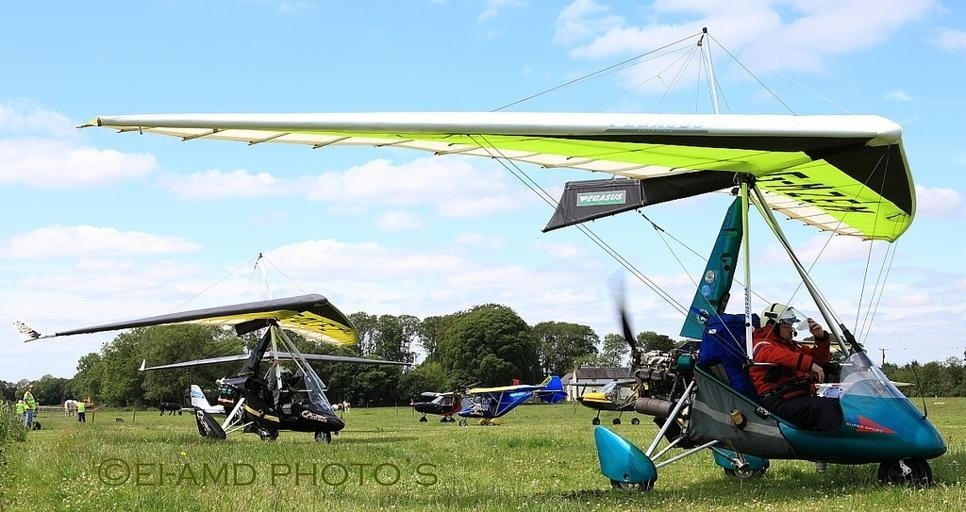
[760,304,801,328]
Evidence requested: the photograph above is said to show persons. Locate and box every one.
[23,385,36,430]
[331,399,351,413]
[267,359,294,414]
[745,302,842,433]
[15,399,24,423]
[76,399,86,422]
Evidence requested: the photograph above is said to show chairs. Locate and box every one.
[698,314,760,402]
